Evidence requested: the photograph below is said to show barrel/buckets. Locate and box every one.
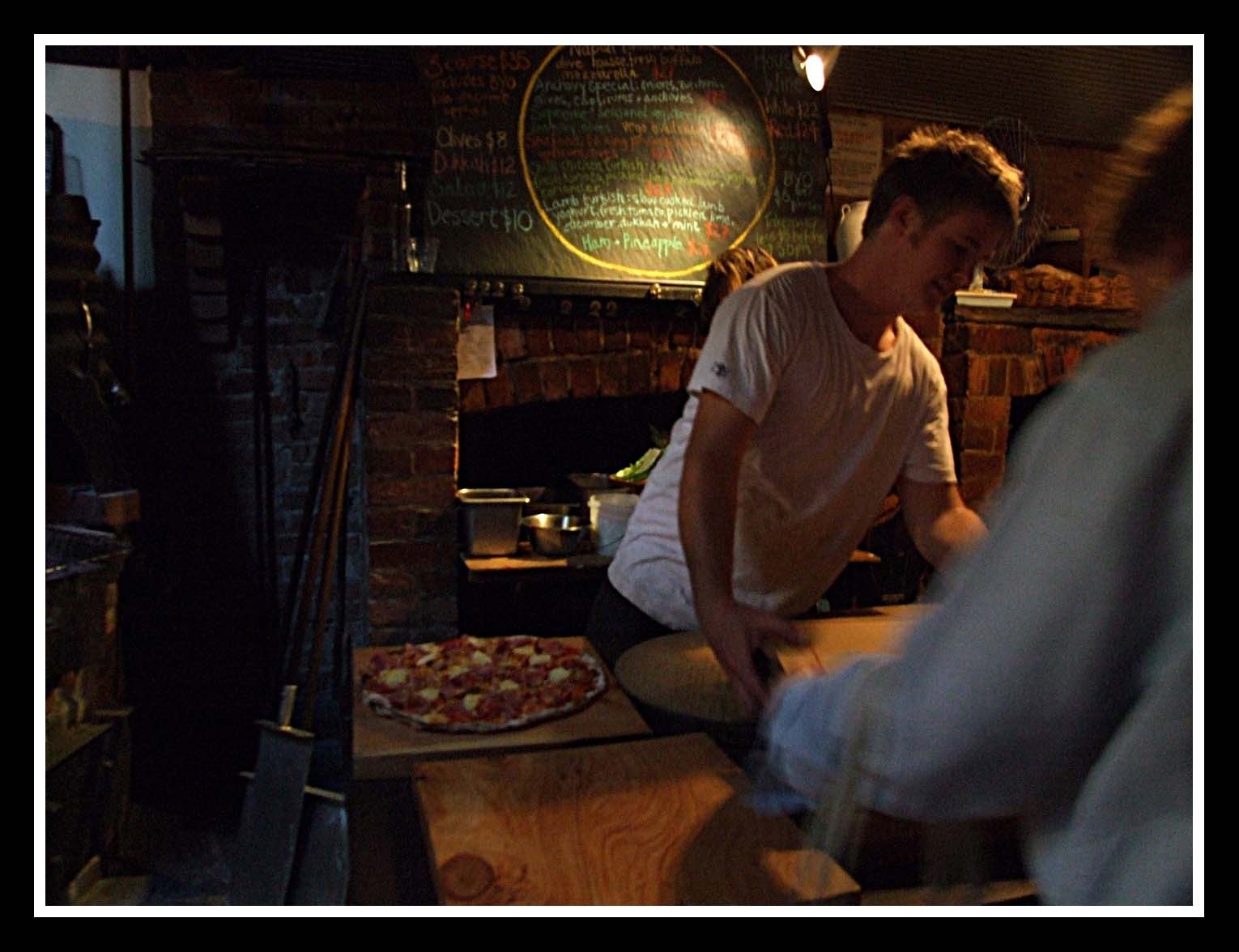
[587,492,639,556]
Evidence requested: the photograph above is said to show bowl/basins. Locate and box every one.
[524,514,590,555]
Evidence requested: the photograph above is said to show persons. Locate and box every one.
[754,76,1193,905]
[589,122,1025,767]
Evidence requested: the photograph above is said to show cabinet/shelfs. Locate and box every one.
[46,526,139,906]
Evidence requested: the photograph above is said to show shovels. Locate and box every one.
[230,187,401,905]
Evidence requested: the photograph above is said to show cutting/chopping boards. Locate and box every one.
[616,631,766,746]
[413,729,867,905]
[349,635,652,782]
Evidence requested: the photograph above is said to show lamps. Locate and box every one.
[793,46,842,92]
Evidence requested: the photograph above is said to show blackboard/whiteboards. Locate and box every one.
[414,45,828,296]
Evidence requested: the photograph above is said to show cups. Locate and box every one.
[408,238,440,273]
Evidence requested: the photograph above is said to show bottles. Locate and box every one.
[388,160,411,272]
[835,201,869,262]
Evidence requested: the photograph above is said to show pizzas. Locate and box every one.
[359,634,608,732]
